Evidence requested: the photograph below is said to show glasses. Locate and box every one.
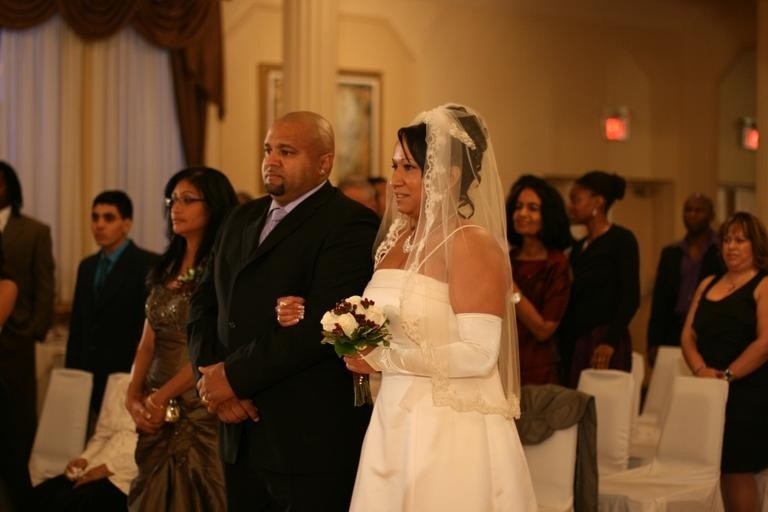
[165,196,203,204]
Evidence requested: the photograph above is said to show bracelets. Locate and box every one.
[694,364,706,376]
[148,396,162,410]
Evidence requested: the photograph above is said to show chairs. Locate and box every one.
[575,368,632,482]
[25,366,95,486]
[597,377,728,509]
[630,350,645,422]
[628,346,694,461]
[96,370,135,425]
[521,422,578,512]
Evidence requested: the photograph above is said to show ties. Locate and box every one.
[258,209,287,245]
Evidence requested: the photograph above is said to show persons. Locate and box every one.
[682,212,768,511]
[366,179,388,222]
[501,176,577,386]
[0,158,56,508]
[646,192,729,373]
[185,110,383,512]
[66,191,162,438]
[126,166,242,512]
[341,103,546,512]
[336,175,379,216]
[13,370,141,511]
[553,171,642,389]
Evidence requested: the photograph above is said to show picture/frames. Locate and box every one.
[254,61,384,199]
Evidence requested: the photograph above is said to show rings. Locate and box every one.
[202,396,208,403]
[277,307,280,315]
[276,315,281,323]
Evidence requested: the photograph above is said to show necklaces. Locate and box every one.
[582,226,609,250]
[402,211,459,255]
[726,270,755,292]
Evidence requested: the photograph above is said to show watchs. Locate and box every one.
[722,369,737,383]
[509,291,524,305]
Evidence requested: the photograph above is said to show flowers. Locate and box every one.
[319,295,390,406]
